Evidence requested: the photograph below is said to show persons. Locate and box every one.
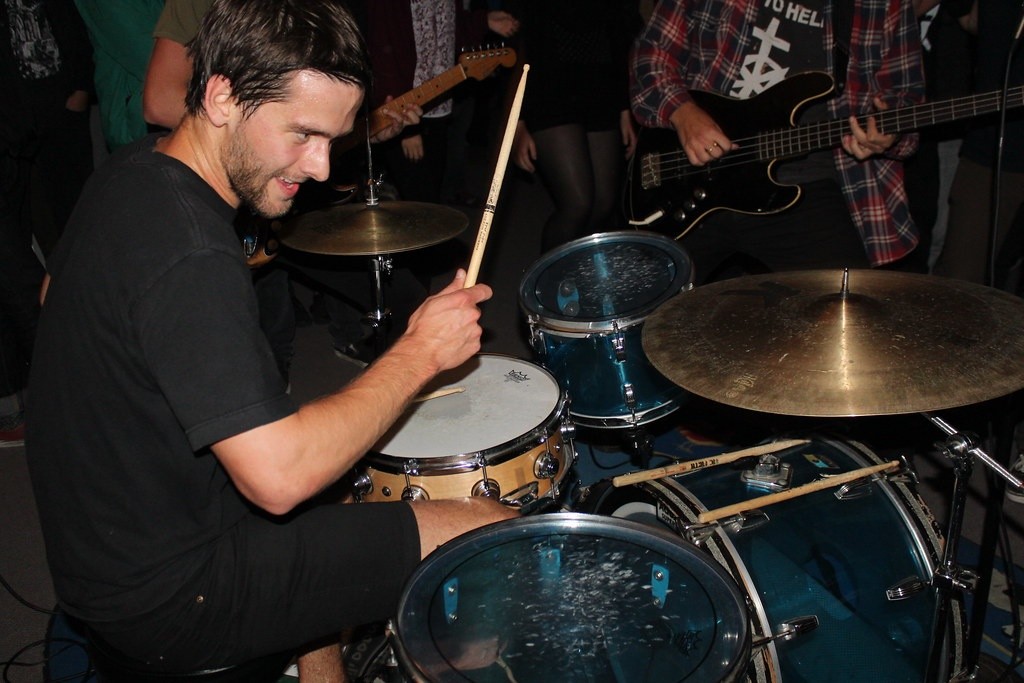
[509,2,638,268]
[629,0,984,289]
[23,1,522,682]
[0,0,519,449]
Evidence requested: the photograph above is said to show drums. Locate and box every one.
[579,432,965,683]
[518,231,701,429]
[346,351,575,514]
[387,512,751,683]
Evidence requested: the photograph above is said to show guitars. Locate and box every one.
[318,41,516,155]
[631,70,1023,247]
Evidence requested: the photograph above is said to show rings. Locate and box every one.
[707,143,718,154]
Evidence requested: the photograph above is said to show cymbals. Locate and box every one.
[273,197,469,256]
[640,268,1024,417]
